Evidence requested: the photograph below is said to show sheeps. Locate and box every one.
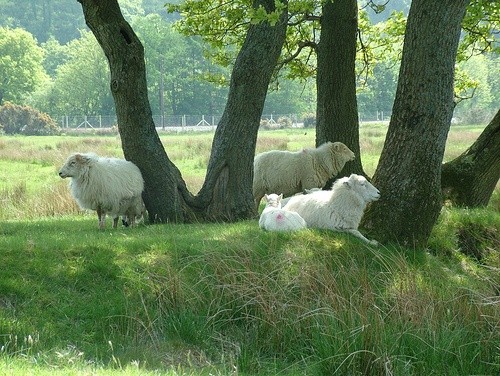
[252,141,357,212]
[59,152,145,230]
[259,173,381,246]
